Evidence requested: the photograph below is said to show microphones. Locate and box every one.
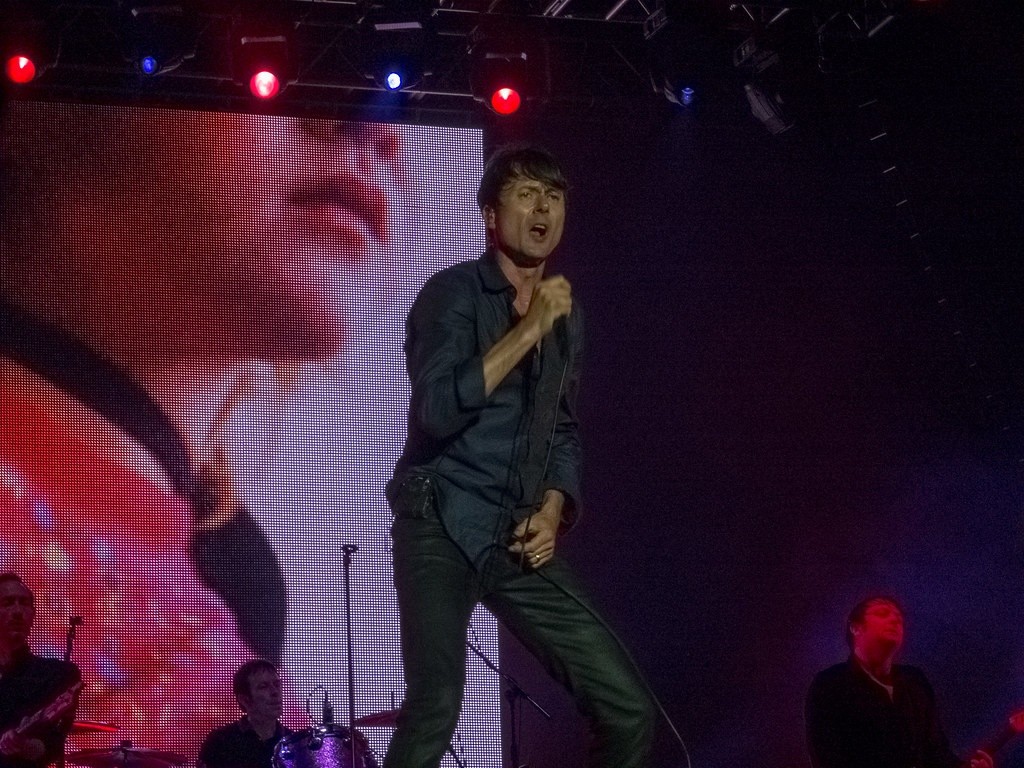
[554,315,569,359]
[322,692,333,723]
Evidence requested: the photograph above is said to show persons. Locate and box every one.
[808,594,998,768]
[198,659,305,768]
[383,143,695,768]
[1,568,88,768]
[0,104,398,768]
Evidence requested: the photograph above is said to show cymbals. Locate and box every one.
[355,708,402,727]
[67,718,121,735]
[68,738,189,768]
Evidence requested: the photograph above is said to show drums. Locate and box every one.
[273,722,379,768]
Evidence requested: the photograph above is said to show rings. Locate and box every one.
[535,554,539,562]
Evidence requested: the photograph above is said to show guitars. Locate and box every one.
[1,680,85,748]
[960,706,1023,767]
